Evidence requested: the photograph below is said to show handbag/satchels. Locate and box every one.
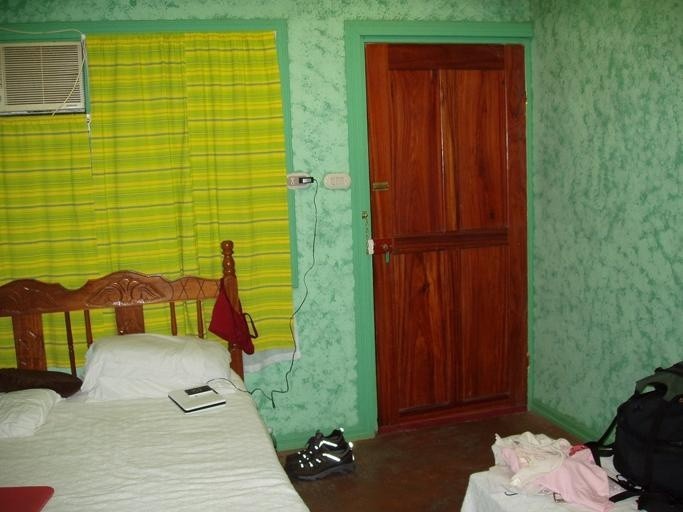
[208,277,255,356]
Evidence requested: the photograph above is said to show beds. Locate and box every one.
[0,239,308,512]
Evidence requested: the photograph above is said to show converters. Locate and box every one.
[299,176,314,184]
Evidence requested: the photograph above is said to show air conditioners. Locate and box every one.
[0,41,85,114]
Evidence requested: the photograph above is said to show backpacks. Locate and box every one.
[613,360,683,500]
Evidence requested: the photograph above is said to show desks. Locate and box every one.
[466,453,641,512]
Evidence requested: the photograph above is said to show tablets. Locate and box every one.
[168,385,226,412]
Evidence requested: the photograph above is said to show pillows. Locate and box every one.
[0,328,232,439]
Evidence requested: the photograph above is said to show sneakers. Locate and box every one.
[283,440,356,483]
[282,424,348,466]
